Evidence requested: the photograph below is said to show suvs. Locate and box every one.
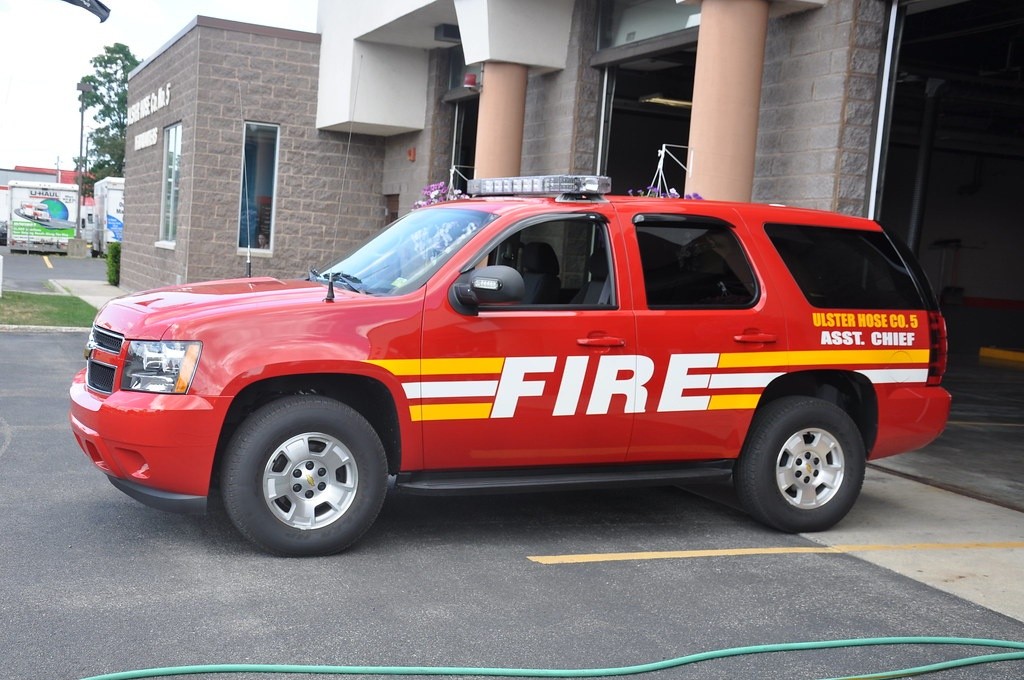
[69,175,951,559]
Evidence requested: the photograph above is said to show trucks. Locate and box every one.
[91,177,125,259]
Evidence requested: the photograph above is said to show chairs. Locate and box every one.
[519,242,562,304]
[572,234,610,305]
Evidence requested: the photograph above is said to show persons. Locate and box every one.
[259,233,269,249]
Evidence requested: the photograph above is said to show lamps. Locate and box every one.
[434,23,461,43]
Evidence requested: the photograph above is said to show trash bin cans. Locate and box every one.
[68,238,88,258]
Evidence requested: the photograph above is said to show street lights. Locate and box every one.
[76,83,93,238]
